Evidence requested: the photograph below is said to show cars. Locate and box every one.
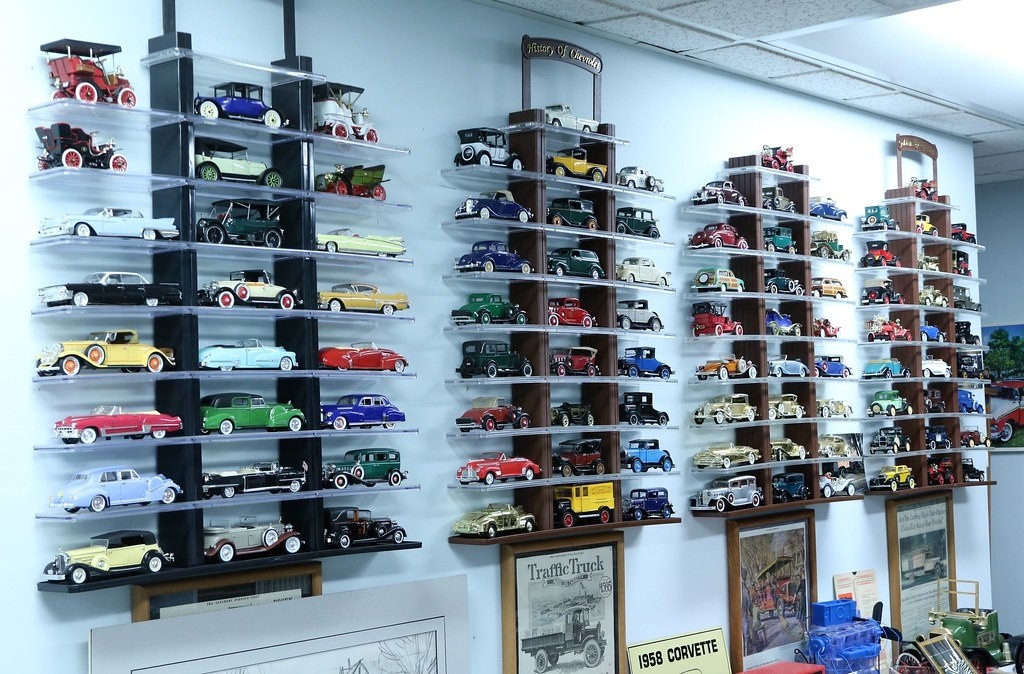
[452,104,676,539]
[194,135,283,190]
[198,269,297,309]
[305,163,390,200]
[36,208,178,241]
[861,182,990,492]
[191,80,283,129]
[35,123,128,172]
[895,606,1024,674]
[40,39,138,105]
[687,149,867,518]
[312,82,379,144]
[38,329,407,583]
[317,283,410,314]
[197,199,284,247]
[991,368,1024,448]
[314,227,407,257]
[37,271,184,307]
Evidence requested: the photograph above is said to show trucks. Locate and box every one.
[901,542,946,587]
[521,605,606,674]
[740,553,808,657]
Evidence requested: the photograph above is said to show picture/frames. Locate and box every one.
[131,560,322,627]
[89,571,473,674]
[884,490,961,667]
[500,533,630,674]
[726,508,818,670]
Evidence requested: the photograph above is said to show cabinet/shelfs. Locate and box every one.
[434,148,679,549]
[27,46,422,593]
[864,187,996,496]
[683,157,862,518]
